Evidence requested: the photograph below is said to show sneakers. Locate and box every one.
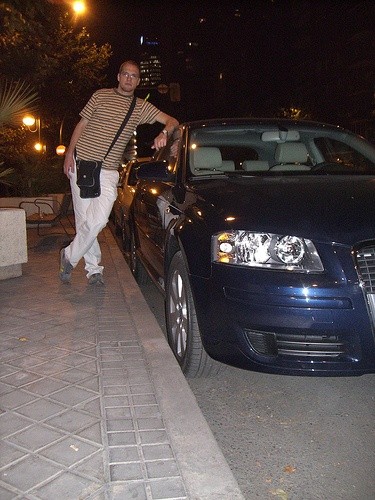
[59,249,73,283]
[88,272,104,287]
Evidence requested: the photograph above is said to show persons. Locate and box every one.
[56,58,181,288]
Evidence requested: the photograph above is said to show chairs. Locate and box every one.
[194,143,310,171]
[19,193,75,243]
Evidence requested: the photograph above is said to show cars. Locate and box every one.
[112,154,162,252]
[127,117,375,382]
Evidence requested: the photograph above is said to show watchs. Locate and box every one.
[160,129,169,138]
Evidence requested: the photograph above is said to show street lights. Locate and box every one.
[61,1,94,23]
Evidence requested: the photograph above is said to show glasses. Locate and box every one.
[120,73,139,79]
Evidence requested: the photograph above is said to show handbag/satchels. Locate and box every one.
[75,159,104,199]
